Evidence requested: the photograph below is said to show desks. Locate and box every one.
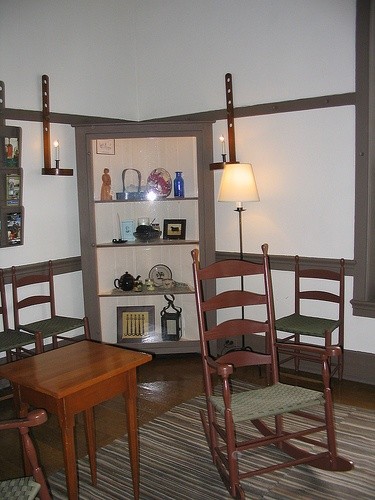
[0,340,154,500]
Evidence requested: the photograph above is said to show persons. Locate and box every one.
[100,168,112,201]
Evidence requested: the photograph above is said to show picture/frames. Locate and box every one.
[117,305,155,344]
[163,219,186,240]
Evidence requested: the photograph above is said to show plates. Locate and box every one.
[149,263,172,286]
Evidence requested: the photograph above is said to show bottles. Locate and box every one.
[152,223,160,240]
[174,171,184,198]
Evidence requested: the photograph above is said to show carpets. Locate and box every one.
[31,380,375,500]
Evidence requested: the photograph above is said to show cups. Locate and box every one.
[133,278,176,291]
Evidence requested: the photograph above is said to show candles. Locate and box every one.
[56,140,59,159]
[221,139,226,153]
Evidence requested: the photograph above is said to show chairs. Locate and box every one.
[0,409,53,500]
[0,269,40,366]
[11,260,91,360]
[191,243,354,500]
[265,255,345,390]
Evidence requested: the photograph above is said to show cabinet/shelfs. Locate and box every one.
[71,122,218,358]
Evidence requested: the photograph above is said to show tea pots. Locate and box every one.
[114,271,141,291]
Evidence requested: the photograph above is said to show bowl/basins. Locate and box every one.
[134,231,162,243]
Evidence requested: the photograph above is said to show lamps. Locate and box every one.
[218,164,260,350]
